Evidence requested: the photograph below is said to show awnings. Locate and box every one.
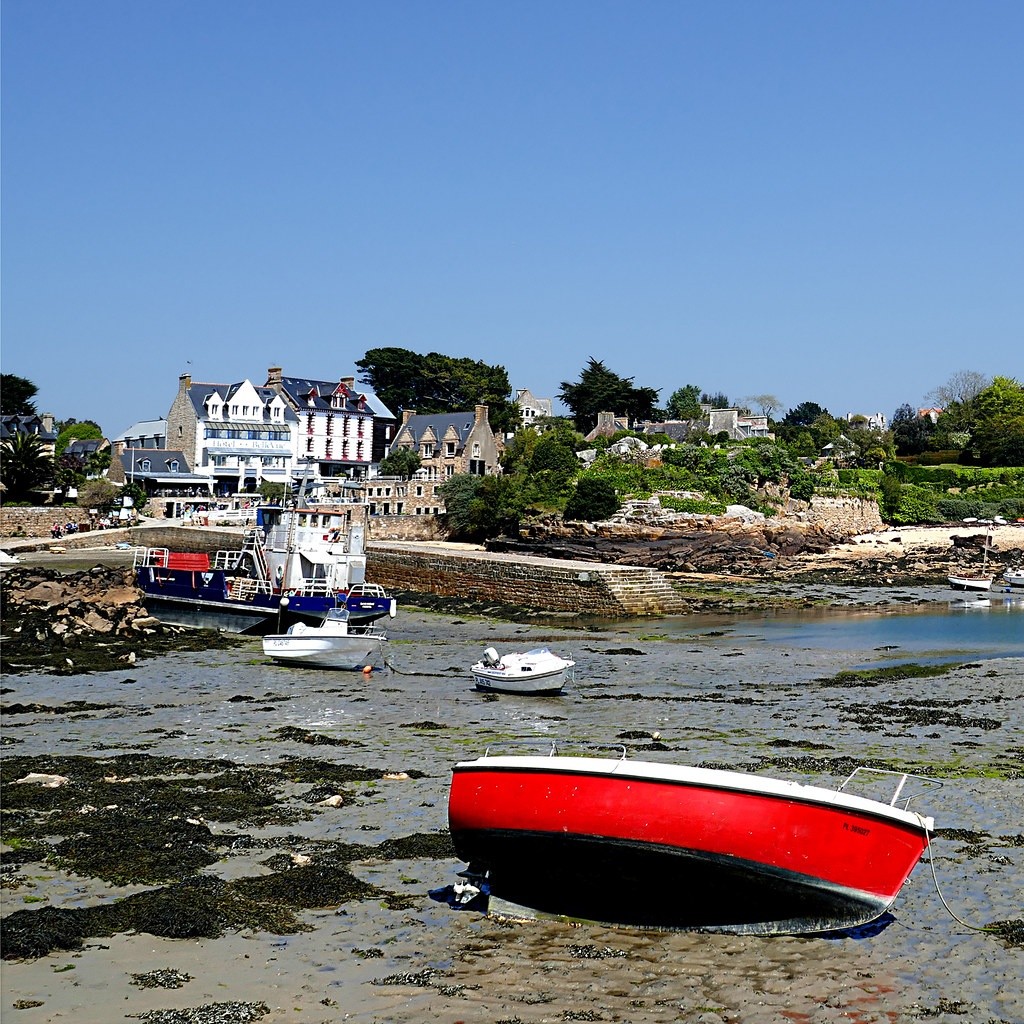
[152,475,297,483]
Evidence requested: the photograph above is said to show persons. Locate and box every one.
[51,486,355,539]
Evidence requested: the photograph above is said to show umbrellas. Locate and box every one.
[303,482,326,496]
[338,482,366,499]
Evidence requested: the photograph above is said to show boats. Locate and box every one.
[470,647,577,695]
[448,731,946,942]
[262,607,389,671]
[1003,569,1024,585]
[132,460,398,624]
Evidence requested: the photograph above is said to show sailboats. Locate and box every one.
[946,527,994,593]
